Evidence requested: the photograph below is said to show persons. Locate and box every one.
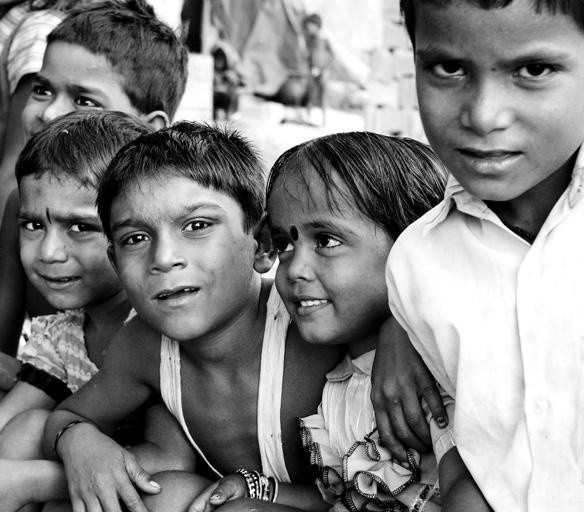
[302,14,334,114]
[0,1,189,510]
[385,1,583,511]
[212,43,240,120]
[189,133,492,512]
[47,120,450,510]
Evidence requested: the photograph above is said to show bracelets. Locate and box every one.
[54,418,101,462]
[236,467,279,503]
